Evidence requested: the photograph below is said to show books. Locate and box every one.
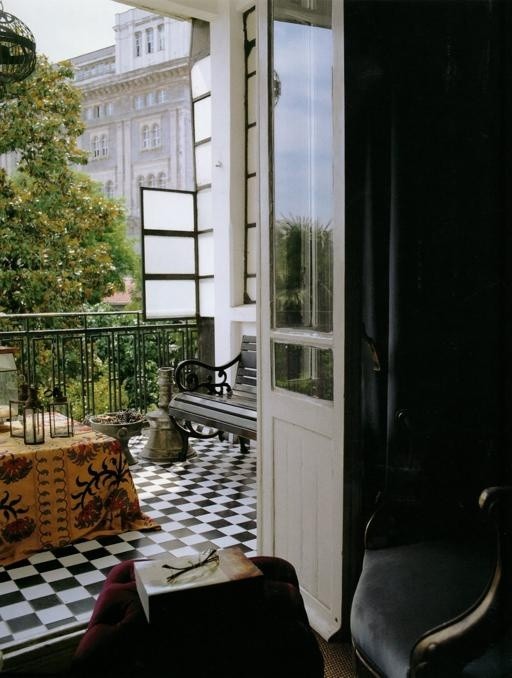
[133,545,266,623]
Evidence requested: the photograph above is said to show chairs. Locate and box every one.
[348,483,511,678]
[68,555,326,676]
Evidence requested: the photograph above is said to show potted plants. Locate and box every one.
[89,411,145,467]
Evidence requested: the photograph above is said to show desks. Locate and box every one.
[0,412,123,564]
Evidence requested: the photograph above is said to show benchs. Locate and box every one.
[168,334,257,463]
